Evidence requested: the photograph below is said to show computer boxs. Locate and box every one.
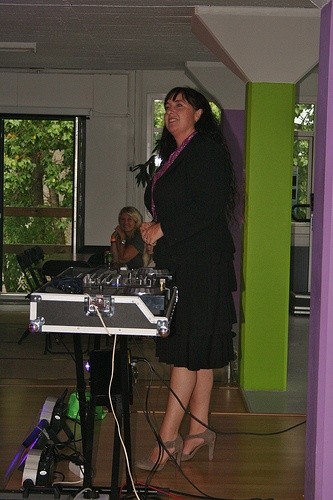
[91,348,134,406]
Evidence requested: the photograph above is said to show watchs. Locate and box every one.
[121,239,126,245]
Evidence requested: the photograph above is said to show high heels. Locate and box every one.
[134,432,183,473]
[168,426,216,462]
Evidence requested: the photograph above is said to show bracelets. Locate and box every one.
[111,236,117,243]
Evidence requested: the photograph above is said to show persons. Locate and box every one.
[110,207,144,270]
[130,87,237,471]
